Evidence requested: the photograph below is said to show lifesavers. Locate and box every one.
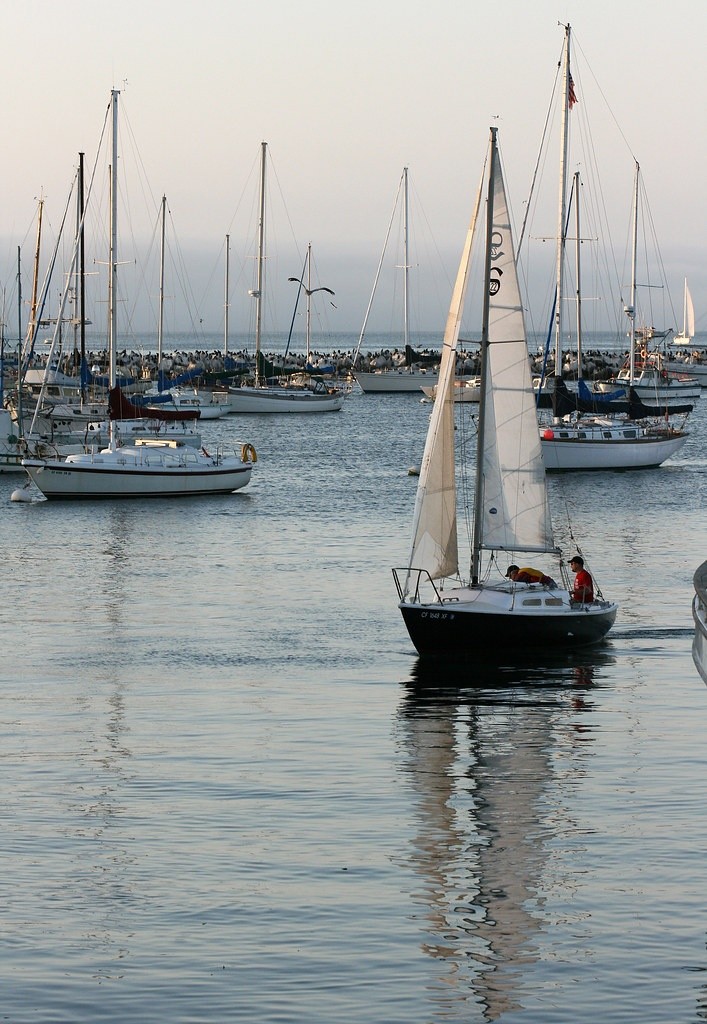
[241,443,258,462]
[641,350,648,358]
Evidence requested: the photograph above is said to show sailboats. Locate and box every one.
[391,124,620,665]
[0,21,706,469]
[20,87,262,502]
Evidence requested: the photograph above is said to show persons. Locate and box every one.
[568,557,594,603]
[505,565,557,587]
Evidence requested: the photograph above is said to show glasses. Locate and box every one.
[571,563,576,565]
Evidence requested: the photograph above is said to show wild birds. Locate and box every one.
[287,277,335,296]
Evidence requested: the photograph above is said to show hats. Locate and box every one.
[505,565,519,577]
[567,556,583,564]
